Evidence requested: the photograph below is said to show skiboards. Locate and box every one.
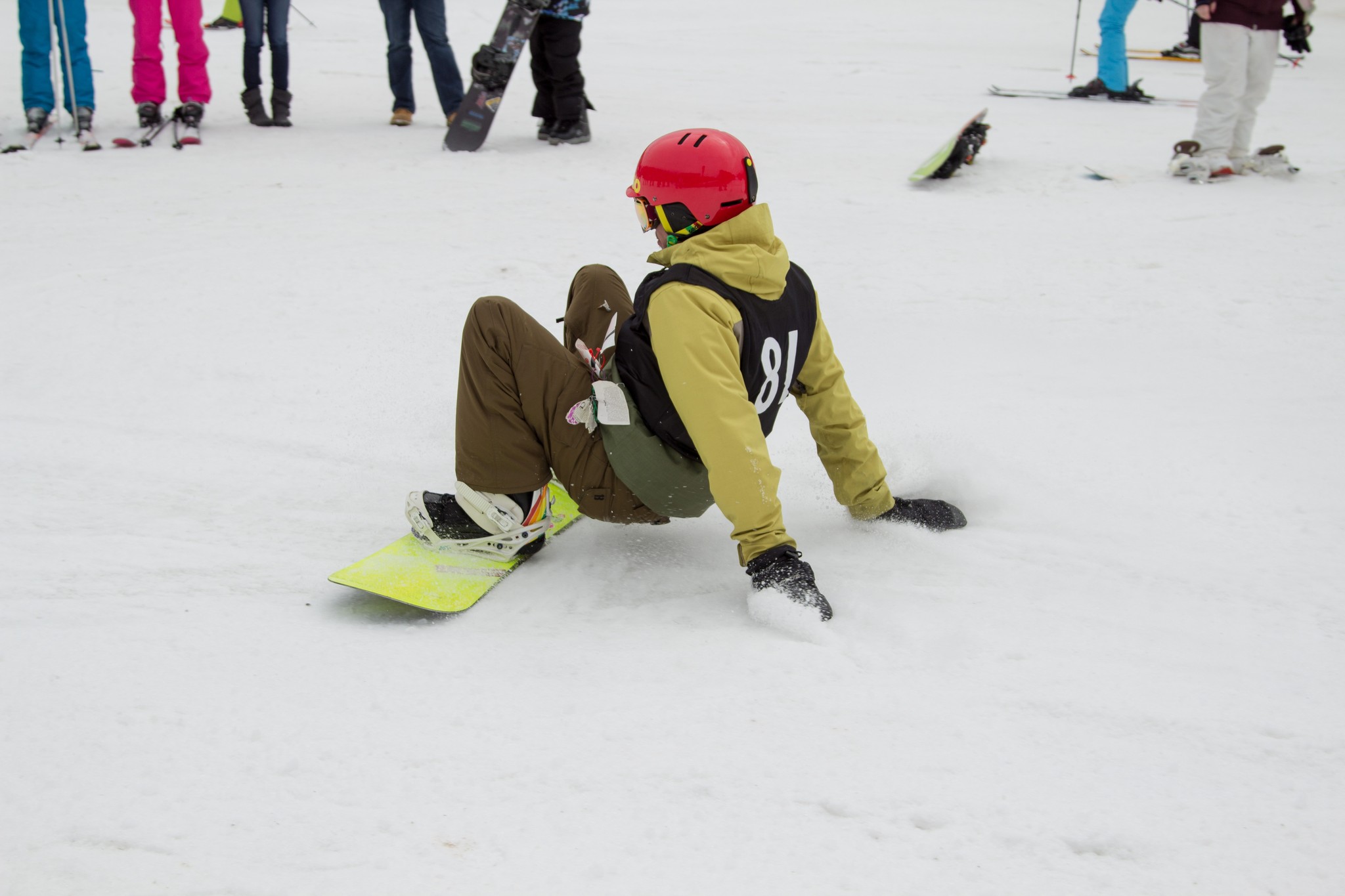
[1080,42,1203,63]
[988,77,1154,105]
[111,104,202,149]
[1,119,102,152]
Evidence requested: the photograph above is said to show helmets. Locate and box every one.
[626,128,758,240]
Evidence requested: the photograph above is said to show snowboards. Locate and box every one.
[326,465,586,616]
[909,106,992,180]
[445,0,554,152]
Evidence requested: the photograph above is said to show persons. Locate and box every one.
[404,128,968,622]
[1193,0,1311,176]
[128,1,213,128]
[1175,12,1200,60]
[529,0,591,145]
[377,0,466,128]
[238,0,292,127]
[1082,0,1139,100]
[209,0,244,29]
[18,0,96,134]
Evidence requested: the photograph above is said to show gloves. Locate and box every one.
[746,545,832,624]
[874,496,967,532]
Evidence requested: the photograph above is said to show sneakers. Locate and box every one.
[1227,151,1254,167]
[406,479,548,556]
[447,111,459,127]
[72,106,93,131]
[1187,151,1233,177]
[390,108,412,126]
[137,103,159,126]
[538,118,557,140]
[179,103,203,127]
[1080,78,1106,94]
[26,107,47,133]
[548,110,591,146]
[1174,40,1200,55]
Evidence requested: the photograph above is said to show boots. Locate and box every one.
[271,89,293,127]
[241,86,274,127]
